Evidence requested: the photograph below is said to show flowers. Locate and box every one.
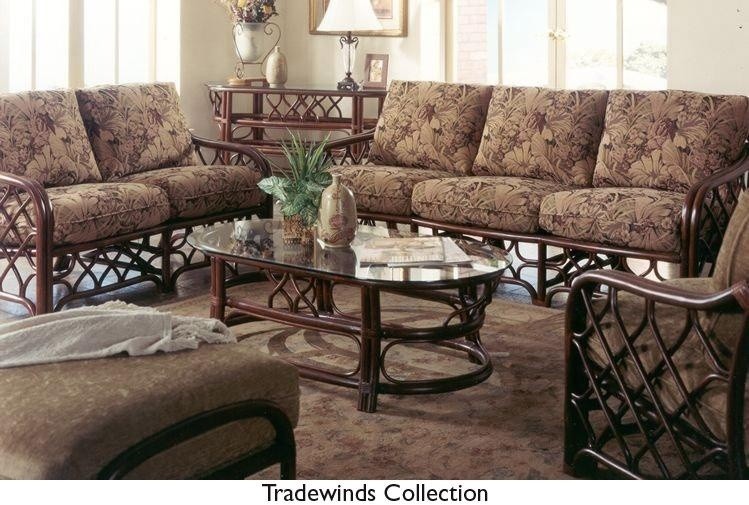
[219,0,278,22]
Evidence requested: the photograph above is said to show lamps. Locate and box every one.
[315,0,382,91]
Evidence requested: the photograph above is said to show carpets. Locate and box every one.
[147,278,568,485]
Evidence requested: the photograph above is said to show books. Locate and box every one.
[356,234,473,267]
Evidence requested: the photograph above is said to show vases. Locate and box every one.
[232,19,289,89]
[316,170,356,247]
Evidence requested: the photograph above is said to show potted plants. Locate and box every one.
[258,130,337,247]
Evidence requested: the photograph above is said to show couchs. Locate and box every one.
[0,82,273,315]
[321,77,749,309]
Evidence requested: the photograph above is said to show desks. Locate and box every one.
[202,84,389,178]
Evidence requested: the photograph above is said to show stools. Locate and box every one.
[0,301,300,478]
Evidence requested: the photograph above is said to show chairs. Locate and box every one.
[562,185,749,479]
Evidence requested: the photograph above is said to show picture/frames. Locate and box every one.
[363,53,389,89]
[308,0,408,38]
